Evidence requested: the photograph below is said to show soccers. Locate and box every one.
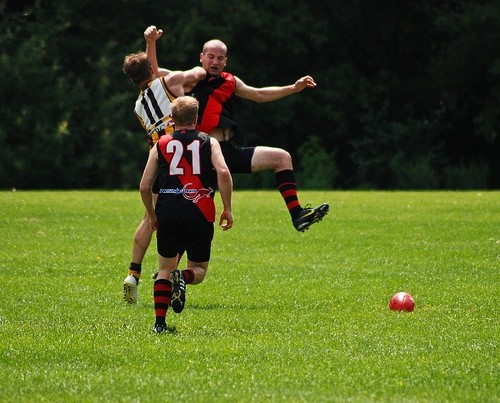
[390,290,417,312]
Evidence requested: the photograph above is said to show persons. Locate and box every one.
[139,97,234,332]
[122,23,329,306]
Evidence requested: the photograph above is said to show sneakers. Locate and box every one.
[169,269,186,314]
[293,203,329,232]
[123,273,138,304]
[153,322,167,335]
